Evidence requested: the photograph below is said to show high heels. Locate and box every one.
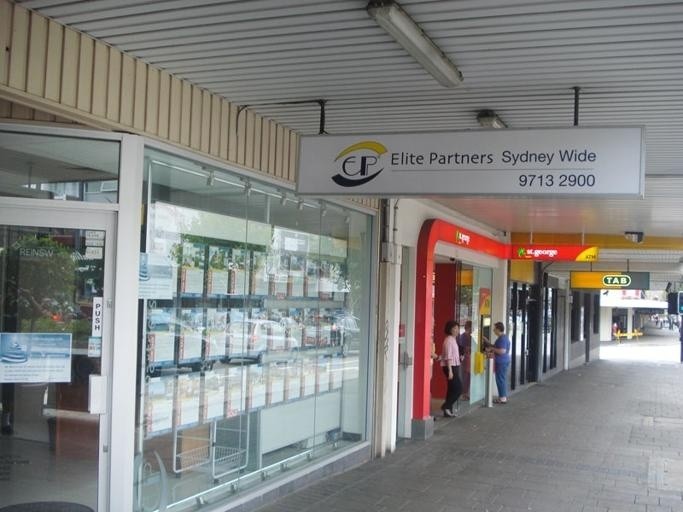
[441,407,455,417]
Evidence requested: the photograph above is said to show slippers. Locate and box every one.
[493,399,507,403]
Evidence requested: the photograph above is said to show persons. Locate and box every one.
[483,322,510,404]
[438,320,463,417]
